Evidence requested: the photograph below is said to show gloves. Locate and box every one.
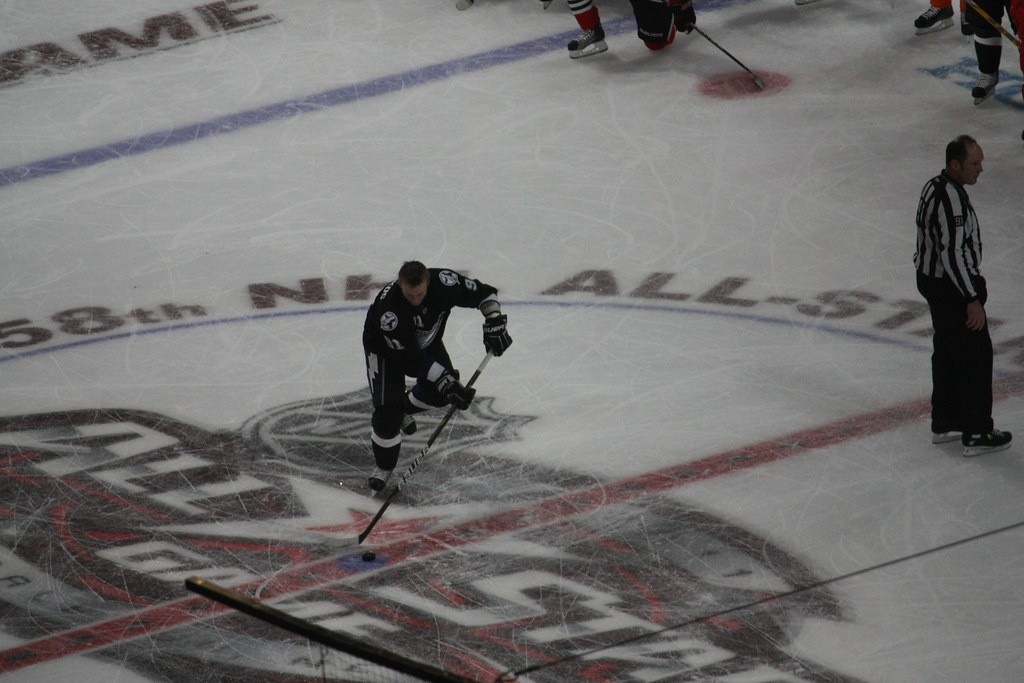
[482,314,513,356]
[672,0,697,34]
[434,368,477,411]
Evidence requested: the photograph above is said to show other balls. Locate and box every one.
[362,552,376,562]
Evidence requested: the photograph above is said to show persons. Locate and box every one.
[363,260,512,499]
[912,0,1024,138]
[566,0,696,59]
[914,134,1012,459]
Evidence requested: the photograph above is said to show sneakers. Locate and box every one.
[931,417,968,434]
[568,22,605,50]
[960,12,974,35]
[914,5,954,30]
[972,71,998,98]
[400,413,418,435]
[368,466,393,492]
[961,429,1012,448]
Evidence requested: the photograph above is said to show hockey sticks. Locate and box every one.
[357,348,496,545]
[690,21,765,88]
[967,0,1019,48]
